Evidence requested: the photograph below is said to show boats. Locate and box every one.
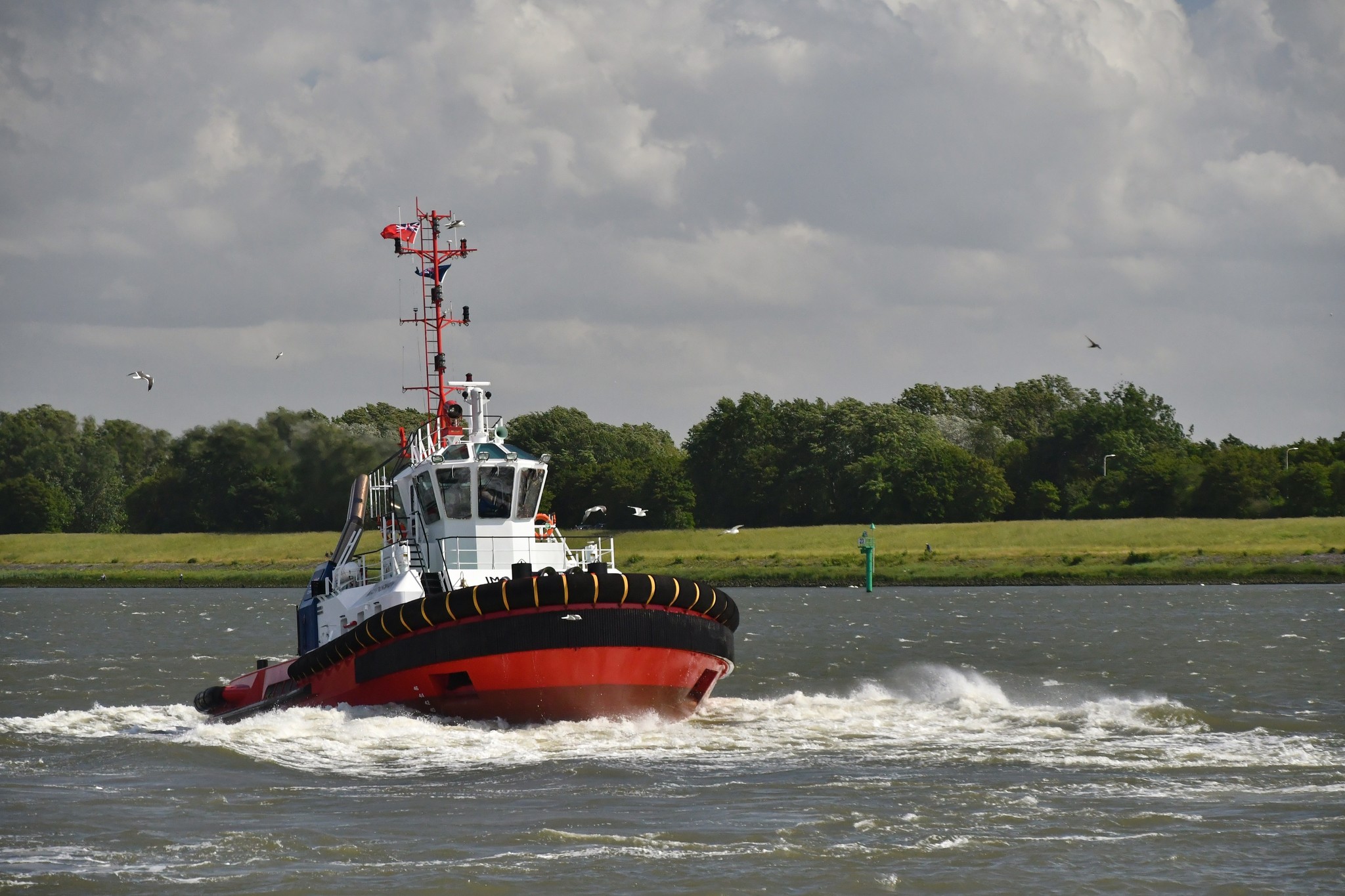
[193,196,739,727]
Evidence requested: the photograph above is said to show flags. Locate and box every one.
[415,264,451,283]
[380,222,420,244]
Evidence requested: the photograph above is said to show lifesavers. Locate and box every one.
[534,513,553,540]
[381,518,407,545]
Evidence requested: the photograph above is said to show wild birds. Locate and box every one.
[925,542,932,552]
[1084,334,1103,350]
[324,551,334,558]
[560,613,583,621]
[275,351,284,360]
[580,505,607,524]
[628,505,649,517]
[716,524,745,539]
[177,573,184,584]
[97,573,107,582]
[125,370,155,392]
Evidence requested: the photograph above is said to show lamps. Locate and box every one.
[431,453,444,464]
[380,476,386,483]
[539,454,551,463]
[506,452,518,462]
[477,451,490,462]
[444,399,463,424]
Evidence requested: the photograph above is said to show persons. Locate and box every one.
[478,467,514,518]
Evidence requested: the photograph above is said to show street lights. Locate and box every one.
[1286,447,1299,499]
[1104,454,1116,477]
